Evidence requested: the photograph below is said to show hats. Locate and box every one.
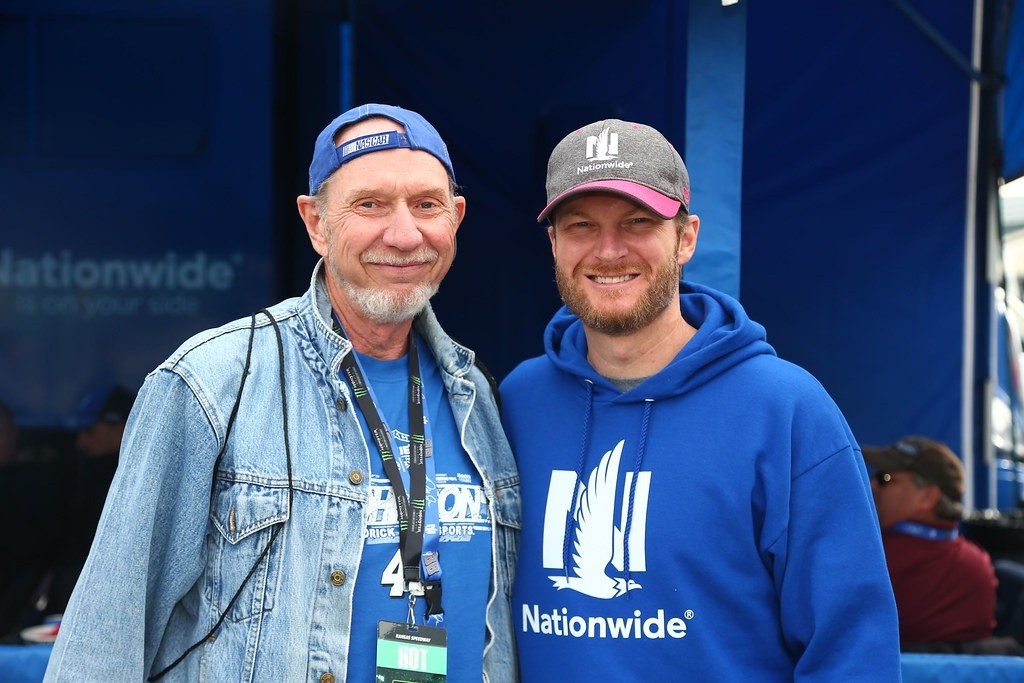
[863,438,962,504]
[309,103,456,193]
[538,118,690,219]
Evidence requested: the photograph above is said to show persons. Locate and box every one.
[861,438,999,650]
[43,104,522,683]
[499,120,899,683]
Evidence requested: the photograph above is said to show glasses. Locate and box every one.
[874,470,926,488]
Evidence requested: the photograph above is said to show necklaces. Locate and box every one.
[895,522,959,540]
